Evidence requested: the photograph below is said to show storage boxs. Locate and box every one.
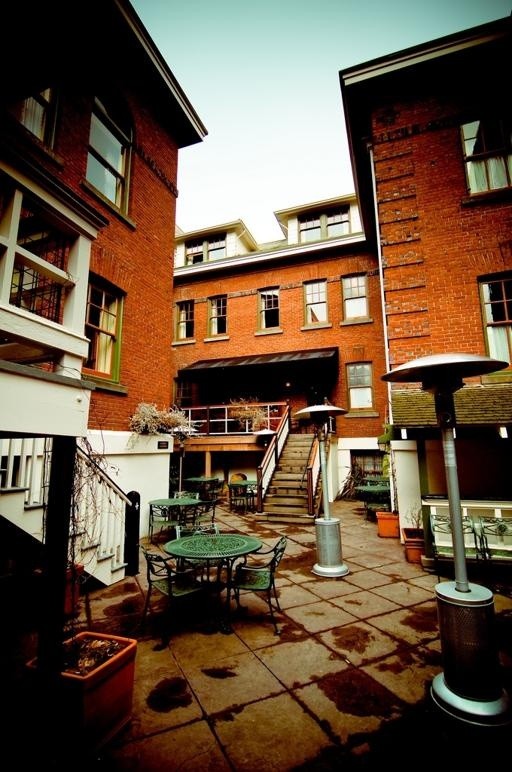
[375,512,400,538]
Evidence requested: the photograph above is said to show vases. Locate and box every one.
[25,630,138,759]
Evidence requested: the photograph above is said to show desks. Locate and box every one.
[363,476,390,486]
[228,481,257,493]
[163,533,262,635]
[354,486,390,505]
[185,477,218,490]
[148,499,202,526]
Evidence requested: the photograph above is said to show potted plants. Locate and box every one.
[401,504,425,564]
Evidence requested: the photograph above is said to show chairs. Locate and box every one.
[430,513,478,583]
[194,498,221,534]
[233,536,288,636]
[228,483,254,515]
[478,515,512,594]
[176,523,223,581]
[174,492,199,524]
[136,544,217,646]
[148,504,178,543]
